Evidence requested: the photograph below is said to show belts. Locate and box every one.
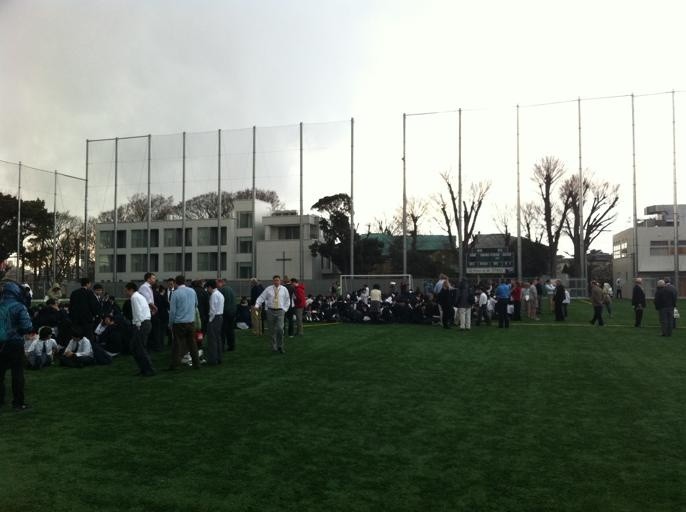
[268,308,282,310]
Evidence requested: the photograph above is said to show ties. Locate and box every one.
[73,342,78,352]
[42,341,46,354]
[274,289,278,310]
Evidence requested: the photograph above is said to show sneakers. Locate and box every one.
[13,404,32,412]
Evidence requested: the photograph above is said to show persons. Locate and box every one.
[0,282,33,409]
[654,280,674,336]
[664,278,677,329]
[632,278,646,327]
